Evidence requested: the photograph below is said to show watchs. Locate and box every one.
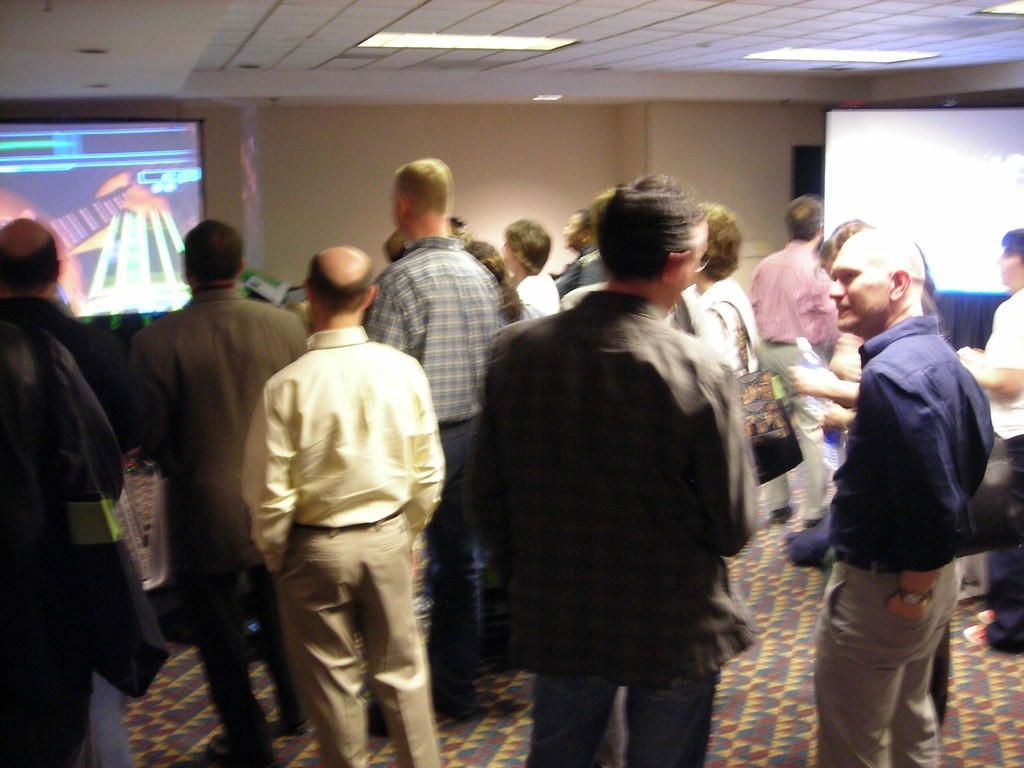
[897,585,925,604]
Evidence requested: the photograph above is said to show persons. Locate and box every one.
[127,156,1024,766]
[0,321,169,767]
[1,219,140,766]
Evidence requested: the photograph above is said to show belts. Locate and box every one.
[294,508,405,535]
[836,549,903,574]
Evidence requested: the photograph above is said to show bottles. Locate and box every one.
[824,426,845,470]
[794,339,826,371]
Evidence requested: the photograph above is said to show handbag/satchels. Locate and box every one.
[703,302,803,488]
[40,325,168,697]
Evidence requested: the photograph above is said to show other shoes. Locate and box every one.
[206,732,236,768]
[365,712,391,739]
[433,681,477,723]
[264,720,310,736]
